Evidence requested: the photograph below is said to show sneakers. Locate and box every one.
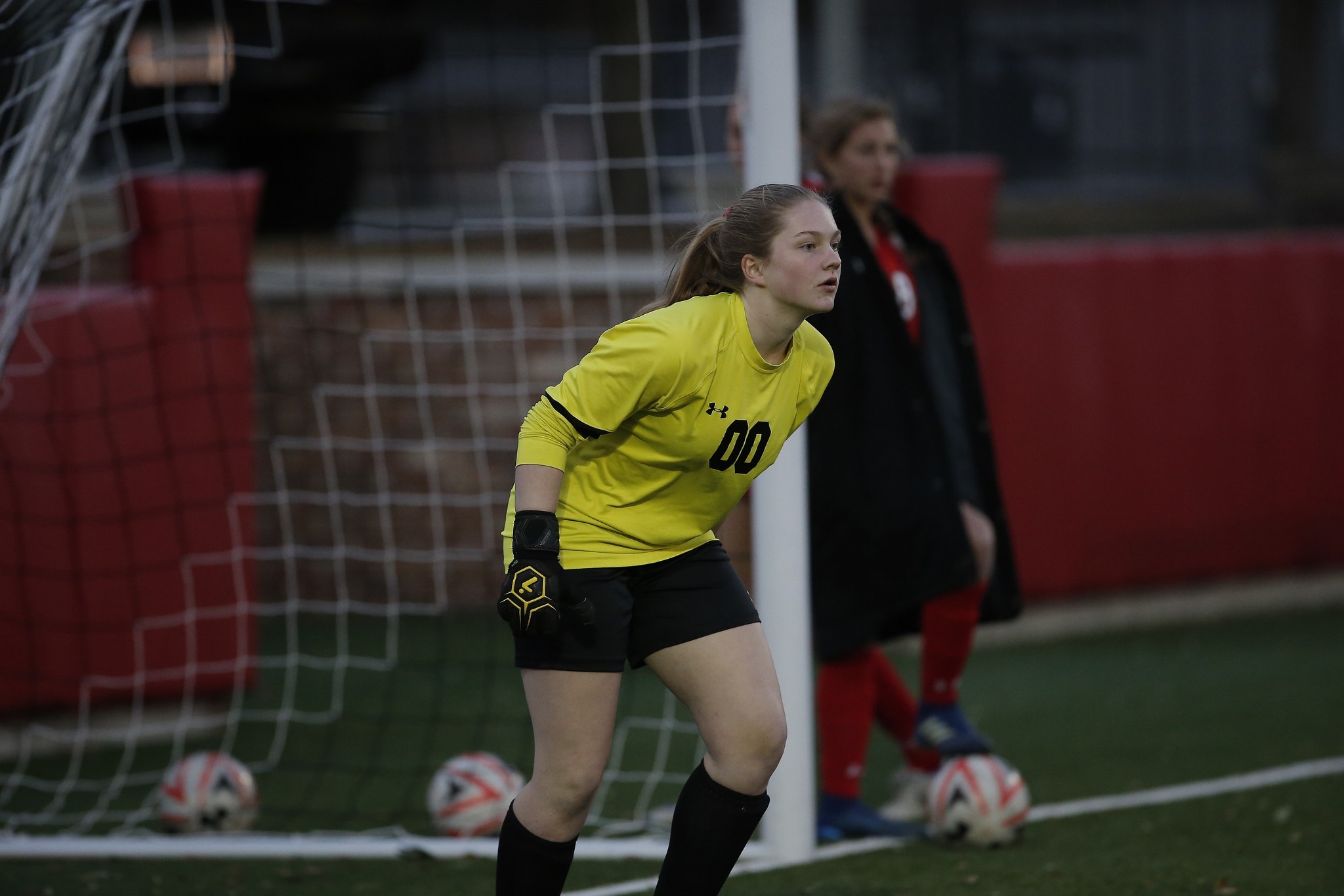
[819,795,921,840]
[913,701,990,757]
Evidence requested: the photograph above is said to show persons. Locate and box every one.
[707,87,946,824]
[488,181,846,896]
[804,89,1025,842]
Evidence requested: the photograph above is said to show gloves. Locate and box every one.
[498,511,569,633]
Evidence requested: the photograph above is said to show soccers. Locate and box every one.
[425,750,523,837]
[156,751,259,834]
[928,756,1027,848]
